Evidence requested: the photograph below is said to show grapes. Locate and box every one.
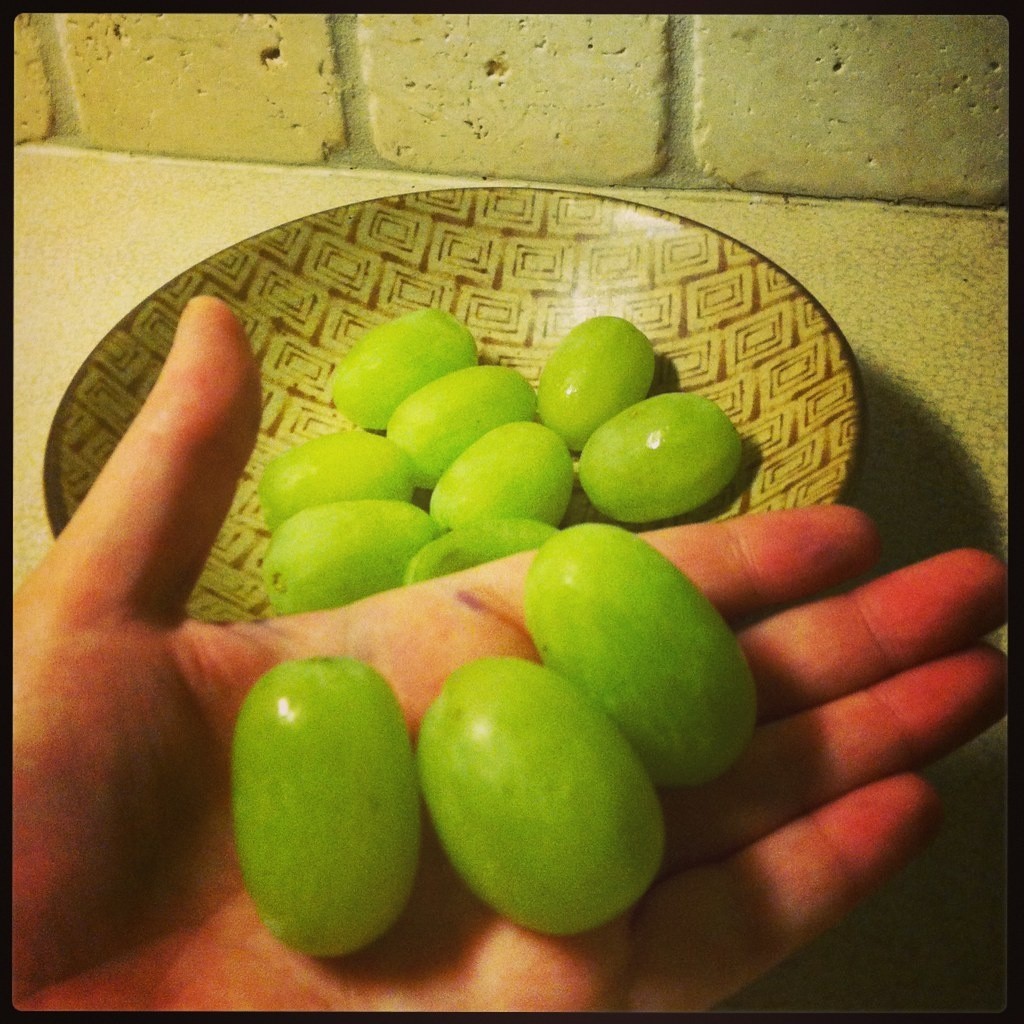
[228,521,758,955]
[250,306,744,624]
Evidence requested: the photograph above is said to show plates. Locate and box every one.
[43,185,859,628]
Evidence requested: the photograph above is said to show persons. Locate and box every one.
[13,295,1009,1011]
